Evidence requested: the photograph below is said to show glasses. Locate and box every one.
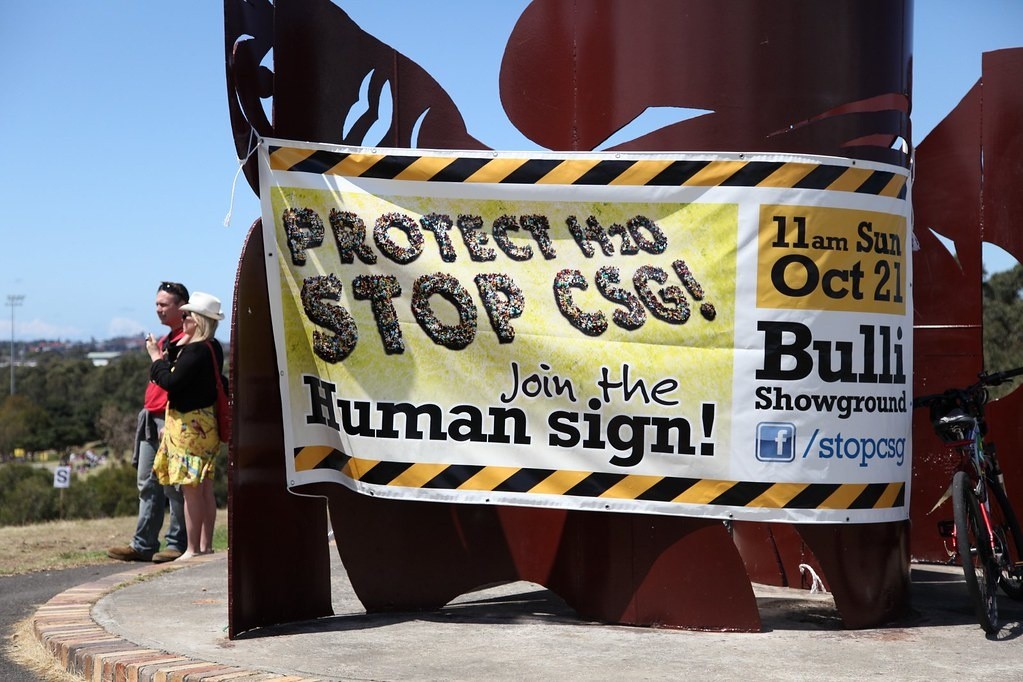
[161,282,184,298]
[182,314,193,320]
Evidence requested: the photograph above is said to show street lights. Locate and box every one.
[4,293,25,397]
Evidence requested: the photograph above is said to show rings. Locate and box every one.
[146,339,148,341]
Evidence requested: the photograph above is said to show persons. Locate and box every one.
[107,282,190,561]
[145,291,225,561]
[59,446,98,474]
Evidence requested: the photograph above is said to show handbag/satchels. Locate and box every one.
[217,393,229,443]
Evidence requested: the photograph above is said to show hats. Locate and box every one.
[179,292,225,320]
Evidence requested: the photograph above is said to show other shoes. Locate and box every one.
[152,549,181,562]
[174,549,215,562]
[107,546,159,562]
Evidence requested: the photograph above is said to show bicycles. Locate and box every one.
[914,367,1023,633]
